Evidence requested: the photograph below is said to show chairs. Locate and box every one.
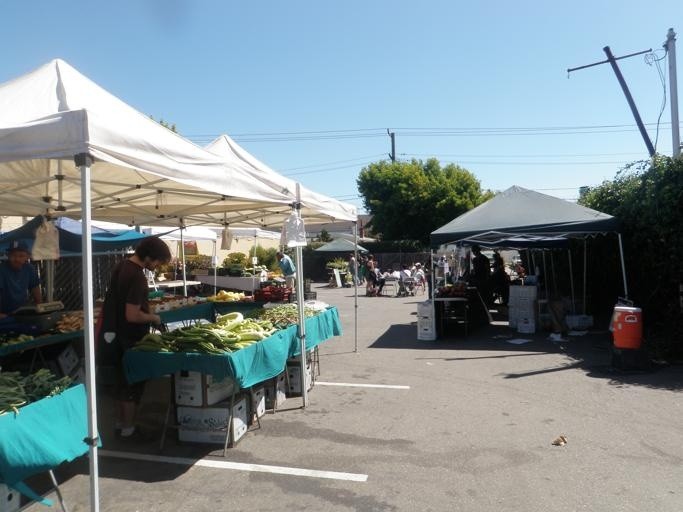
[374,269,424,297]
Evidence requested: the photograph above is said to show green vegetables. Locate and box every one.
[23,368,72,396]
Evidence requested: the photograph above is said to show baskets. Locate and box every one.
[255,286,317,303]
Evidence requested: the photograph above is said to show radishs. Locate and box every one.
[238,329,271,340]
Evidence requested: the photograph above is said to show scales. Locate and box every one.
[14,301,64,315]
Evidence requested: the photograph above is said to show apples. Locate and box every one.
[263,285,292,300]
[437,283,465,296]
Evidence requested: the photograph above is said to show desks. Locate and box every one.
[0,377,86,510]
[125,305,338,456]
[0,292,252,355]
[429,297,469,338]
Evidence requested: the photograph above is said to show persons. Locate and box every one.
[469,246,491,315]
[437,254,458,277]
[424,254,440,299]
[100,237,171,445]
[276,252,296,290]
[349,253,426,296]
[489,249,504,273]
[1,238,41,318]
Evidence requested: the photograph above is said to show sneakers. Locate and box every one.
[115,424,156,444]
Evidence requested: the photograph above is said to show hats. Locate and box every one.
[5,240,28,251]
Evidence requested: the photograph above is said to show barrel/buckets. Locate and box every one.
[612,306,643,349]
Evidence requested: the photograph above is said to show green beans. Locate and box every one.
[0,370,36,414]
[244,305,305,319]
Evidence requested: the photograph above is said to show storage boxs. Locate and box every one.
[41,345,80,377]
[67,366,85,386]
[177,393,249,448]
[174,366,249,407]
[250,356,314,423]
[0,483,20,509]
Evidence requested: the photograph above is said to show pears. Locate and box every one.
[207,290,245,302]
